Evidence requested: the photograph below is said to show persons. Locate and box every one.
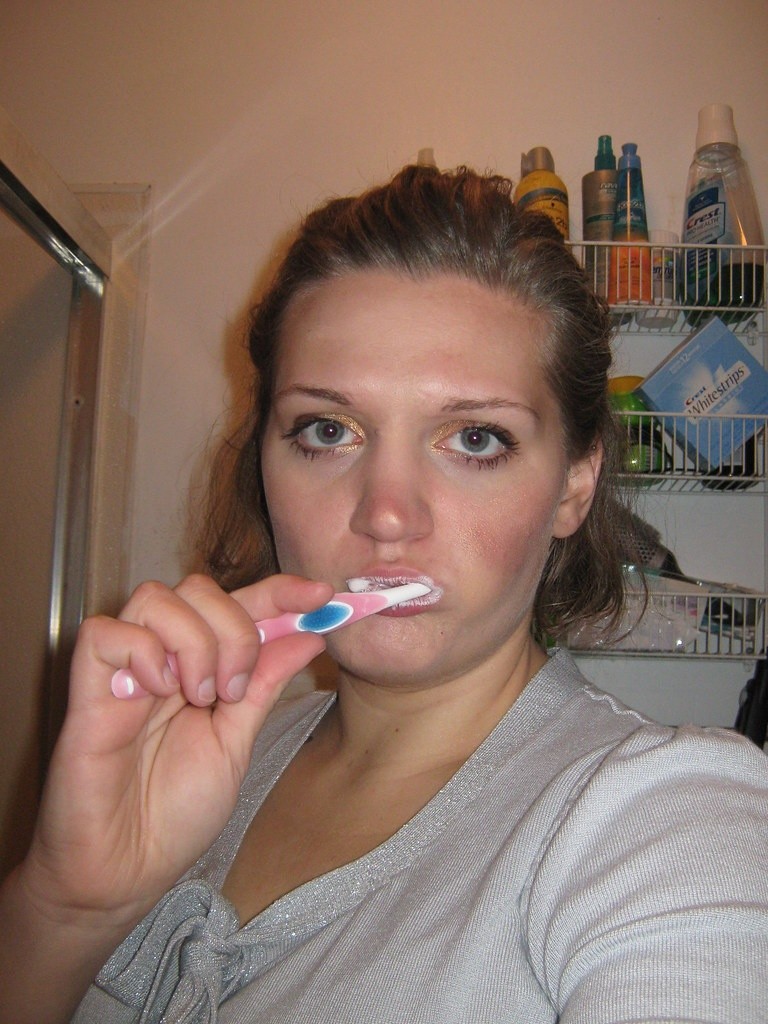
[0,164,768,1024]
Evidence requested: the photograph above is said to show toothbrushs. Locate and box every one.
[108,583,433,703]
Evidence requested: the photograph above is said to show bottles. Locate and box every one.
[609,143,652,305]
[637,230,681,328]
[514,147,569,243]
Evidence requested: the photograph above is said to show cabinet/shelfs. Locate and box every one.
[552,237,768,659]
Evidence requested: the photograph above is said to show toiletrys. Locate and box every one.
[678,102,766,324]
[512,133,654,316]
